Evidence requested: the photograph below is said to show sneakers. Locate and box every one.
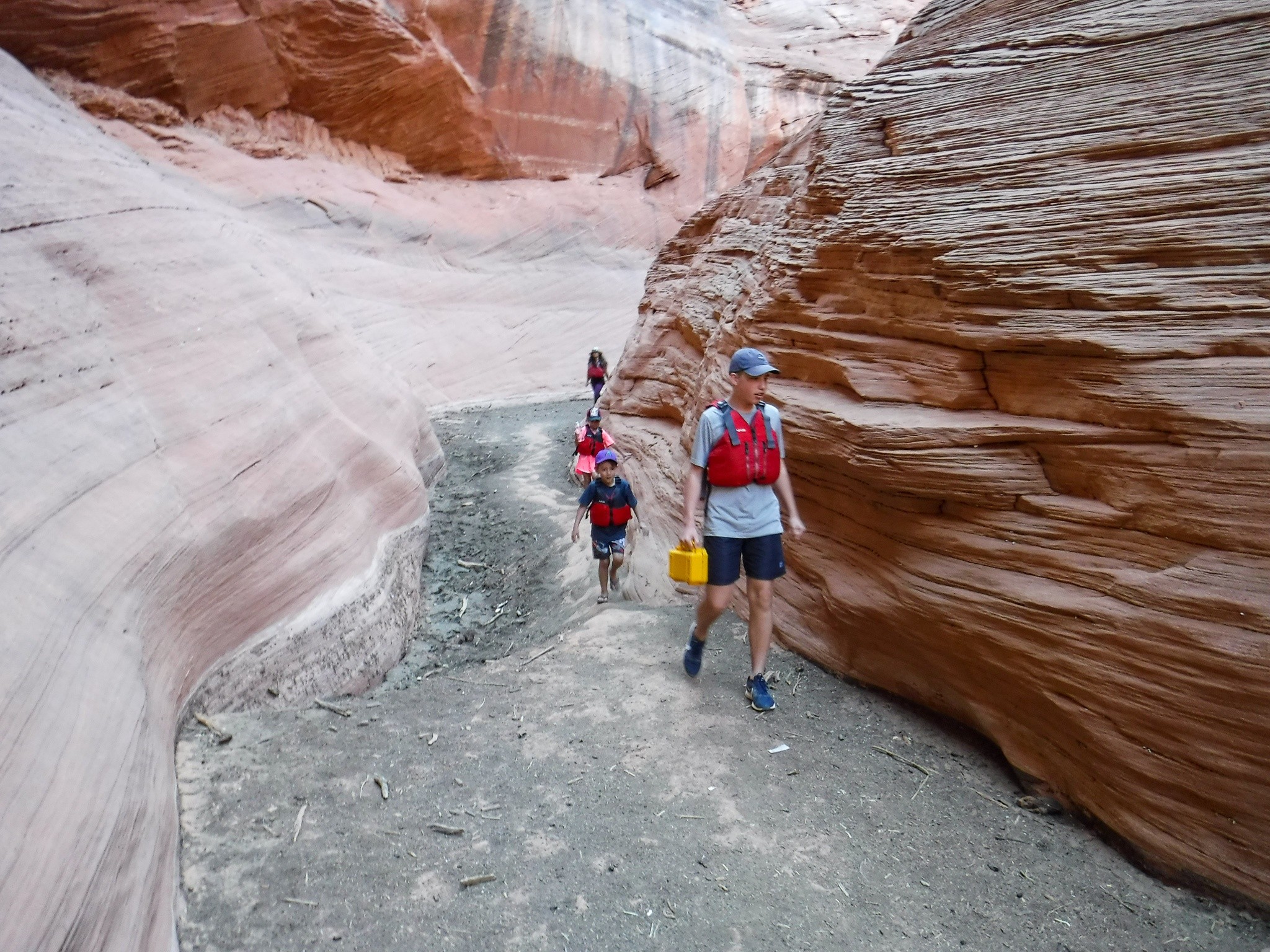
[683,622,706,678]
[745,673,775,711]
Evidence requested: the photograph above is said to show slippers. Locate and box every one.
[597,592,608,604]
[610,573,620,592]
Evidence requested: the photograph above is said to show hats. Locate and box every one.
[587,407,601,421]
[729,348,780,376]
[595,449,618,464]
[589,347,602,355]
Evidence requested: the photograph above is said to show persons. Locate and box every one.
[585,346,609,404]
[679,347,807,710]
[575,406,633,509]
[571,449,649,604]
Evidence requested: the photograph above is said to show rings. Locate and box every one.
[572,536,573,538]
